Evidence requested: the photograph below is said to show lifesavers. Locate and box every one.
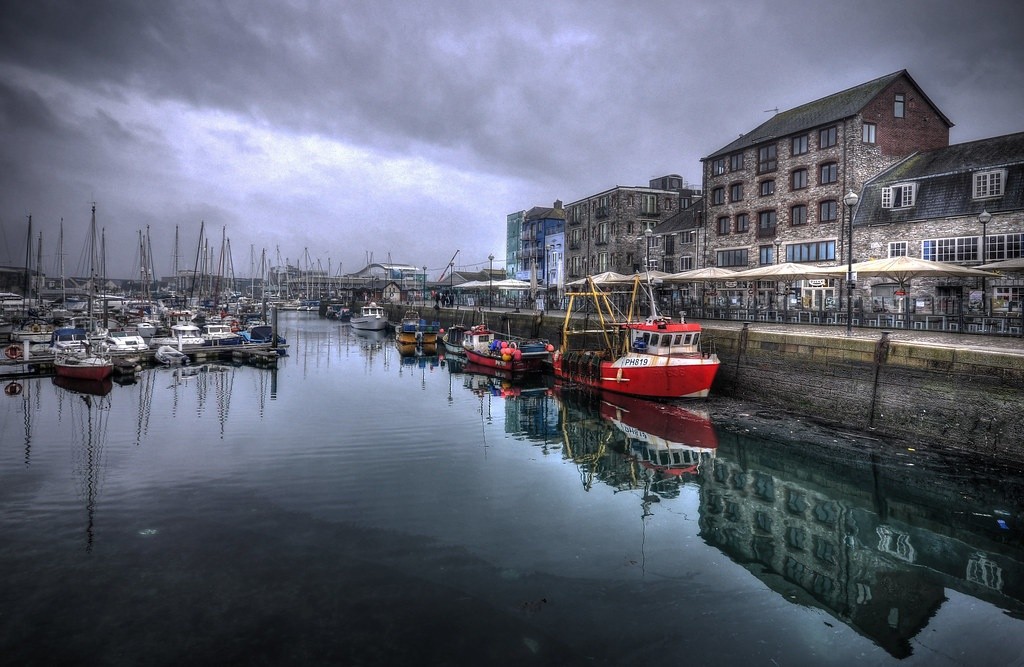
[508,342,517,356]
[4,344,24,359]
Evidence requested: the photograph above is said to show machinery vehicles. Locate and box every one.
[438,249,460,282]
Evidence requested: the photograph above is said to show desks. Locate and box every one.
[459,292,1024,334]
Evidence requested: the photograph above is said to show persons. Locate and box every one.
[431,289,455,308]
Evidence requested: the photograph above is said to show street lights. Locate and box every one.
[385,271,388,303]
[841,189,861,336]
[546,245,551,314]
[450,262,454,308]
[644,229,652,318]
[977,209,993,316]
[488,255,495,310]
[423,266,427,306]
[400,268,403,304]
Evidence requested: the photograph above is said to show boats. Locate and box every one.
[442,324,468,355]
[462,361,542,398]
[54,376,113,397]
[461,325,550,371]
[394,309,439,356]
[350,302,388,330]
[546,377,718,575]
[154,347,190,366]
[552,265,720,398]
[54,340,113,381]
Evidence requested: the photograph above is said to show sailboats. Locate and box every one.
[0,202,286,355]
[286,248,320,310]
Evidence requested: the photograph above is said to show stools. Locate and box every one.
[459,300,1024,337]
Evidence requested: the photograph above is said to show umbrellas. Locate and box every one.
[453,278,541,289]
[564,254,1024,287]
